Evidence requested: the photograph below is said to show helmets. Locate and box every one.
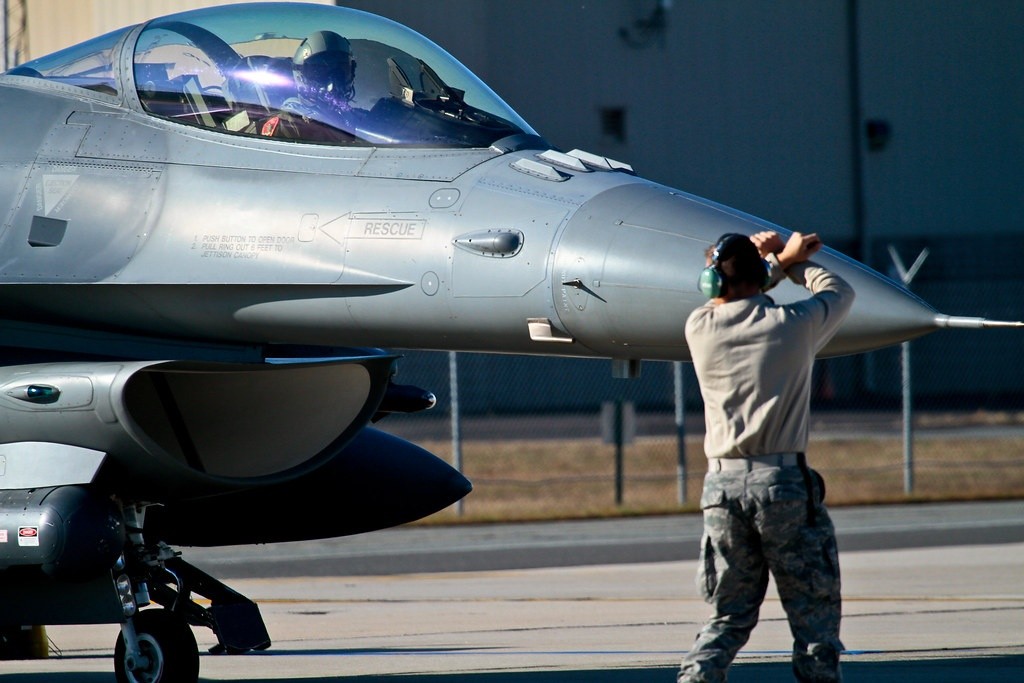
[292,30,354,96]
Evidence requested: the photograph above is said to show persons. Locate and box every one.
[675,229,858,683]
[263,29,358,145]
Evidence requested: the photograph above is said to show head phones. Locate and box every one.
[697,232,773,298]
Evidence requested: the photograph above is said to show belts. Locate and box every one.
[708,452,807,471]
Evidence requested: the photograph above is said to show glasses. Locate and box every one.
[303,62,353,83]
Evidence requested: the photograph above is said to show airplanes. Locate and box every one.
[0,2,1024,683]
